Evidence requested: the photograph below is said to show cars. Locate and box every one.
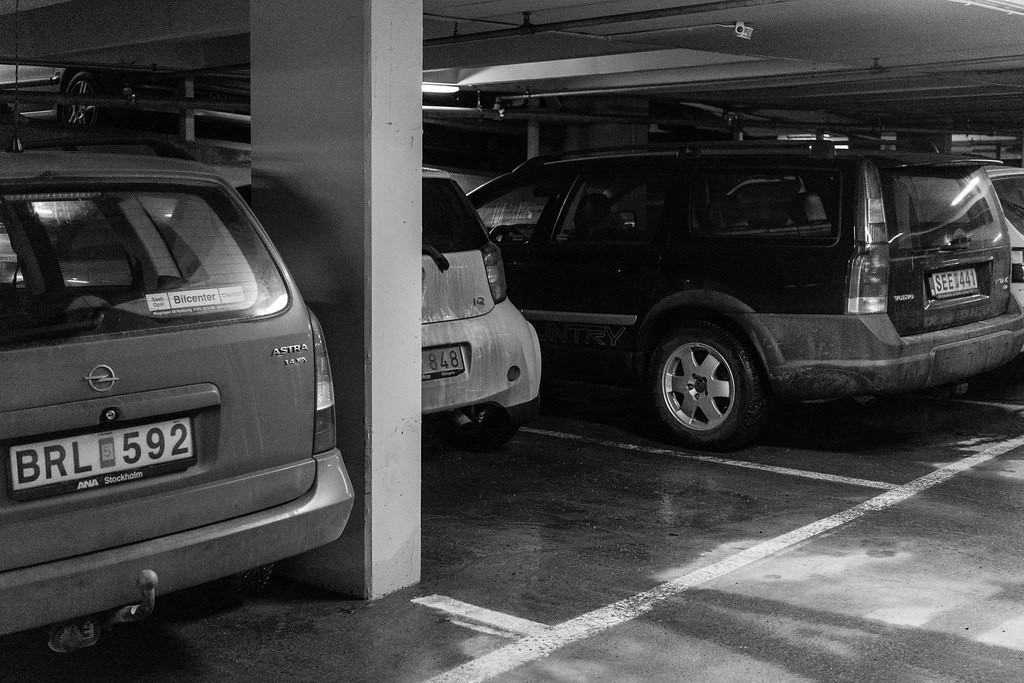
[422,166,542,454]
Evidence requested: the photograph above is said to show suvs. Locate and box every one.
[0,65,355,654]
[464,140,1024,451]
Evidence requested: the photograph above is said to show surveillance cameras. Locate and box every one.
[735,22,754,40]
